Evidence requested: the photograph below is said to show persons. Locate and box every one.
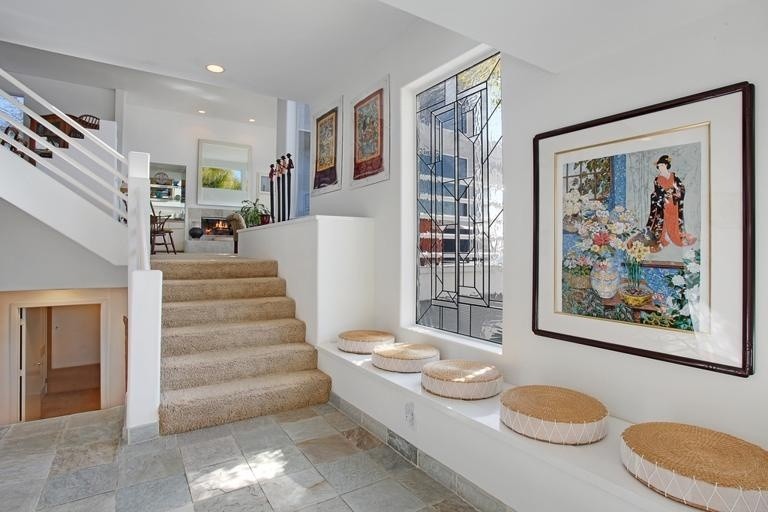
[626,154,696,253]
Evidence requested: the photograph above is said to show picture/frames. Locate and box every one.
[531,80,756,378]
[308,71,390,198]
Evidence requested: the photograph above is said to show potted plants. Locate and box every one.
[257,208,271,224]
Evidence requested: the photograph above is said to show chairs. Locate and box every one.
[150,215,177,255]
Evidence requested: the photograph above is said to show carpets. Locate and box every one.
[150,248,332,437]
